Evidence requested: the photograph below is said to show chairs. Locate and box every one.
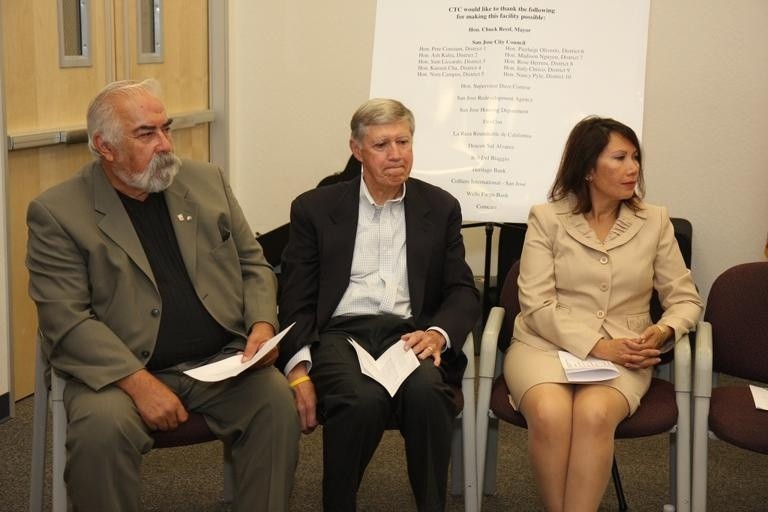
[477,217,691,511]
[691,261,768,512]
[30,329,235,511]
[450,332,478,511]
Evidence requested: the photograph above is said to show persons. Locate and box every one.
[24,77,298,510]
[503,113,706,512]
[270,96,481,508]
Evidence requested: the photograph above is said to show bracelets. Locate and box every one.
[290,376,310,389]
[656,325,667,347]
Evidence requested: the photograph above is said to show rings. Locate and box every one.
[426,346,434,353]
